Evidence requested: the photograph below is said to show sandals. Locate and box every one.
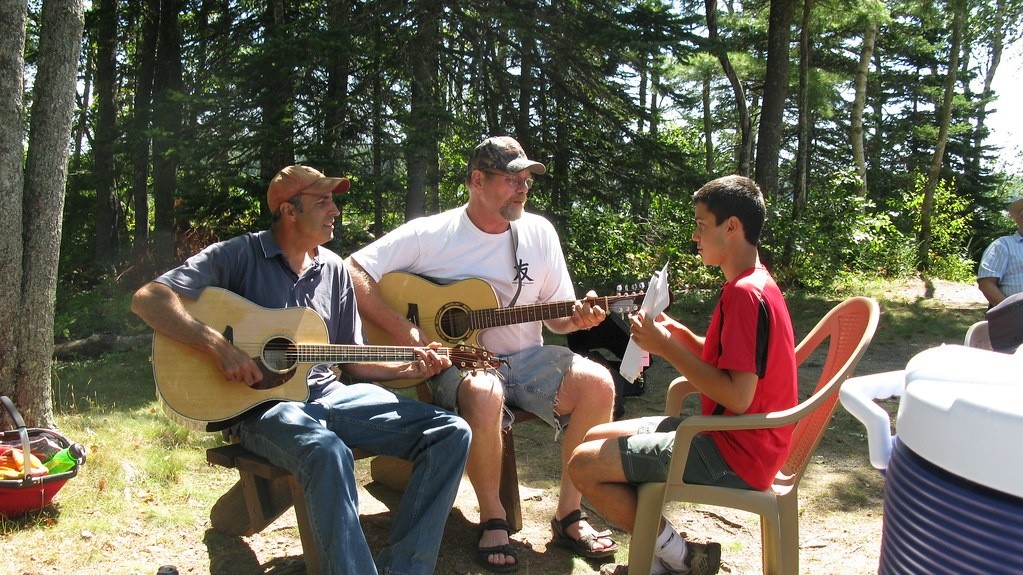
[550,509,619,558]
[475,518,519,572]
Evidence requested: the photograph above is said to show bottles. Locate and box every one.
[43,442,85,475]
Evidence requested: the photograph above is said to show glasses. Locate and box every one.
[475,169,533,188]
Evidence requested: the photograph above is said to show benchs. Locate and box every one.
[203,403,543,575]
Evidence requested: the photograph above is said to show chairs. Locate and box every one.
[964,319,994,352]
[627,296,880,575]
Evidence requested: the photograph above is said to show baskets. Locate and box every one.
[0,396,80,517]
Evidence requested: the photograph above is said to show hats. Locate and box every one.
[266,165,350,214]
[1007,199,1023,224]
[468,136,546,174]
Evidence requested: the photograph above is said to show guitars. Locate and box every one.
[359,272,672,359]
[151,286,504,422]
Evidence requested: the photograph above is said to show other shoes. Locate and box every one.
[600,562,628,575]
[659,541,722,575]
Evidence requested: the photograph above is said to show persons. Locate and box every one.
[131,166,472,575]
[345,136,619,572]
[977,197,1023,307]
[568,175,799,575]
